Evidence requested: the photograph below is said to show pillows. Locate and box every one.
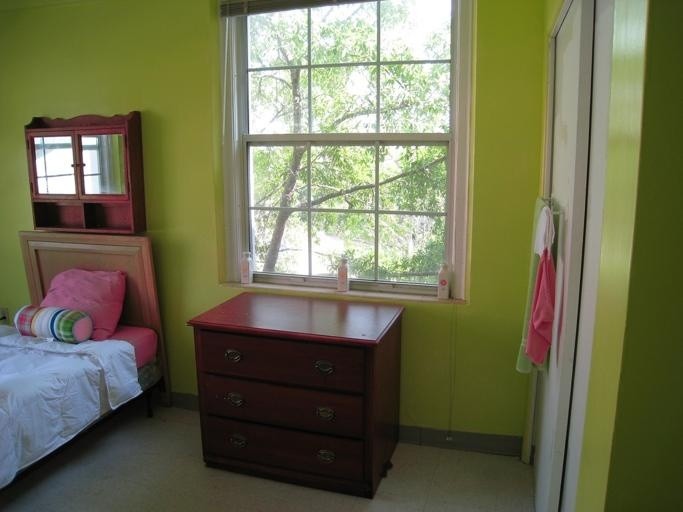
[13,268,126,345]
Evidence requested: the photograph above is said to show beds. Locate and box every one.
[0,232,171,507]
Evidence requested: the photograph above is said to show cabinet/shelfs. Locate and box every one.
[22,110,148,236]
[186,291,403,498]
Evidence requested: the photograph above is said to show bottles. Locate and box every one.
[240,252,252,284]
[337,258,348,292]
[438,262,449,300]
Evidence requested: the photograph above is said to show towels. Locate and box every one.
[515,196,557,376]
